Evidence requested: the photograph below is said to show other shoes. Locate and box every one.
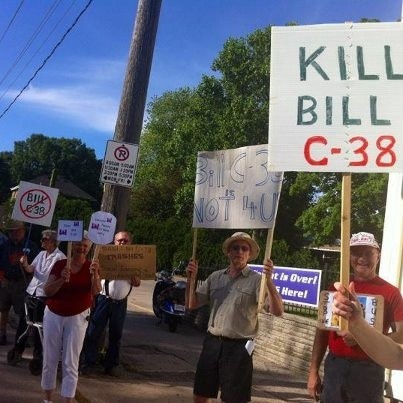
[0,334,7,346]
[104,366,114,374]
[16,354,22,361]
[80,365,94,374]
[43,400,52,403]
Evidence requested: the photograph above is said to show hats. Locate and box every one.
[350,232,379,249]
[222,232,260,262]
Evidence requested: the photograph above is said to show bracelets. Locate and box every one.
[93,275,99,280]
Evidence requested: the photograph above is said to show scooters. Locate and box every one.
[152,260,188,332]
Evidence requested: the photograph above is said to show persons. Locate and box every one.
[7,230,66,376]
[185,231,283,403]
[0,221,41,346]
[80,231,140,376]
[307,232,403,403]
[41,230,102,403]
[331,281,403,370]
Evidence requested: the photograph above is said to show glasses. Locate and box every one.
[41,237,48,243]
[351,250,374,259]
[230,245,250,252]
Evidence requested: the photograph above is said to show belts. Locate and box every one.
[99,295,127,304]
